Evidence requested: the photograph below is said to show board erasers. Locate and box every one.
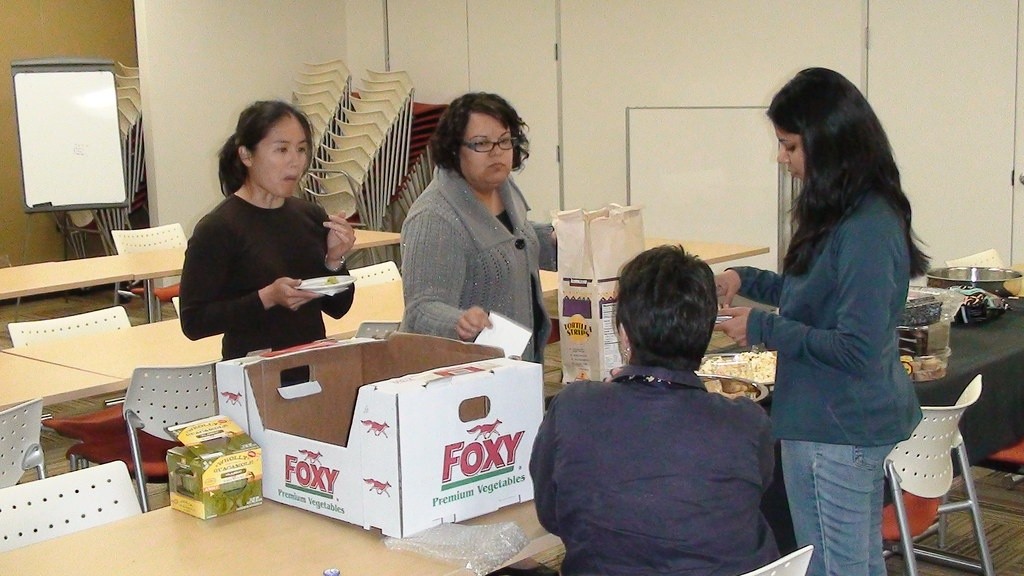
[32,202,53,207]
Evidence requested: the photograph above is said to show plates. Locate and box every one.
[716,316,733,322]
[294,276,357,296]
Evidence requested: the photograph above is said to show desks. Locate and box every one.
[1,268,561,486]
[0,231,401,351]
[1,295,1024,575]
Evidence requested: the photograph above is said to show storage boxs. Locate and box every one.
[166,414,263,519]
[214,329,544,536]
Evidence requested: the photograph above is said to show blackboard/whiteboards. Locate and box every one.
[625,106,782,275]
[12,58,131,213]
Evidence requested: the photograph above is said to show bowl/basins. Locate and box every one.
[927,267,1023,298]
[899,347,951,381]
[695,374,775,402]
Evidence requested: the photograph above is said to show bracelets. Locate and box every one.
[322,252,346,273]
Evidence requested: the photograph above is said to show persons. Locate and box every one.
[401,92,559,372]
[713,67,931,576]
[180,99,356,360]
[530,244,780,576]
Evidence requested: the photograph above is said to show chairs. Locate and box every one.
[286,55,456,280]
[0,360,220,555]
[937,434,1024,548]
[881,374,996,576]
[9,61,188,347]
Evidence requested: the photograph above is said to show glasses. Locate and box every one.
[462,131,518,153]
